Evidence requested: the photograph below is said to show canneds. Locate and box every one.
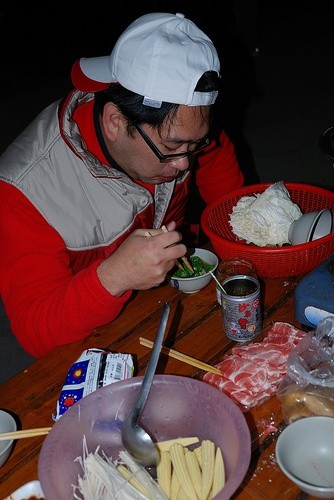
[216,257,262,342]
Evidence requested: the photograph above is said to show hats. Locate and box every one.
[71,12,221,106]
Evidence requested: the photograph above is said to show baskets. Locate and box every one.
[200,181,334,280]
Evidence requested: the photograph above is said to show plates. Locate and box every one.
[4,480,46,500]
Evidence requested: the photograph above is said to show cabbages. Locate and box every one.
[228,182,303,248]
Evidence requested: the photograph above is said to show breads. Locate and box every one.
[282,383,334,423]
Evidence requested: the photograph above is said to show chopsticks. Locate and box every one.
[0,427,52,441]
[144,225,195,273]
[139,336,223,376]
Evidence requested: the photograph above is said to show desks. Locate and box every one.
[0,242,322,500]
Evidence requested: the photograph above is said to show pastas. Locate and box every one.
[115,436,227,500]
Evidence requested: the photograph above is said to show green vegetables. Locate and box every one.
[170,256,214,278]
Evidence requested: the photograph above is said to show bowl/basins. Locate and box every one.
[0,409,17,468]
[287,209,332,246]
[275,416,334,499]
[165,247,218,293]
[38,375,251,500]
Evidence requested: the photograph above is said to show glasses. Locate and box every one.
[135,112,216,162]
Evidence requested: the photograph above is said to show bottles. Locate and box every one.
[216,257,258,306]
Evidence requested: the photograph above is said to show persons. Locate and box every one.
[0,12,245,389]
